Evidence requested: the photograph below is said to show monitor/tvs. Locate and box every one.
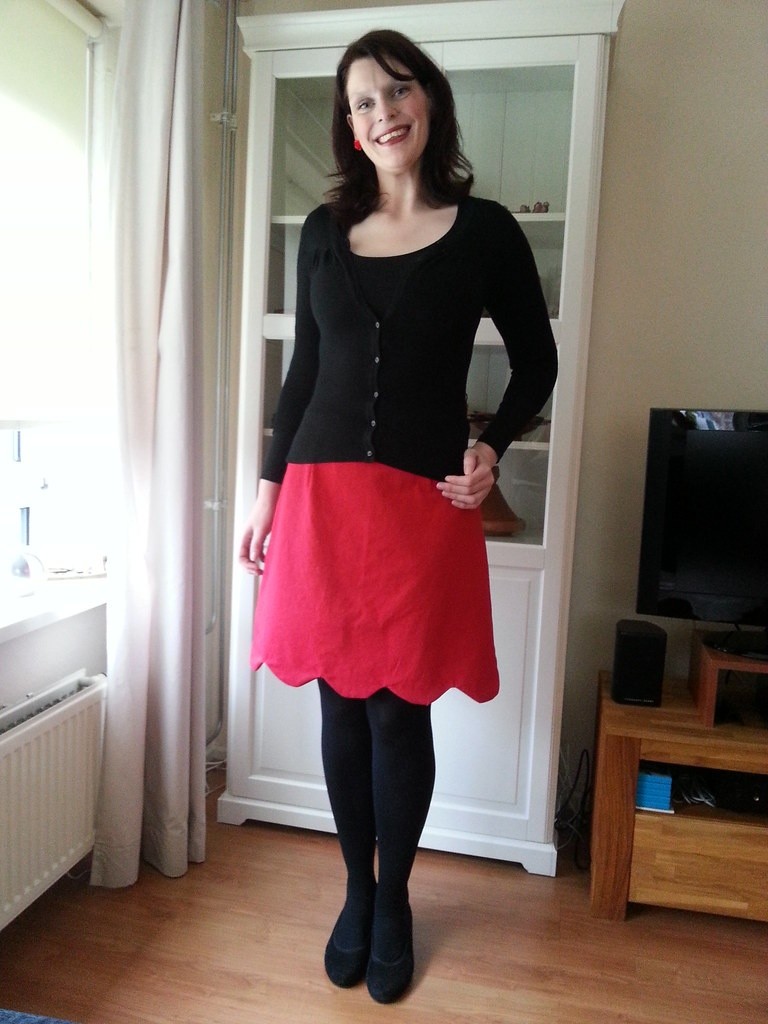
[637,407,768,660]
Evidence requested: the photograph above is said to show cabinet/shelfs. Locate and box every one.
[217,0,627,877]
[588,629,768,921]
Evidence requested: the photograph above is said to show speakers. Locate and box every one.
[612,619,668,707]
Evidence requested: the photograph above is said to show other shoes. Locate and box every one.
[325,911,372,989]
[368,898,413,1005]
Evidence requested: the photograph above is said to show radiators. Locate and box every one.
[0,669,108,935]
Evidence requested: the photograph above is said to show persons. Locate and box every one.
[240,30,559,1005]
